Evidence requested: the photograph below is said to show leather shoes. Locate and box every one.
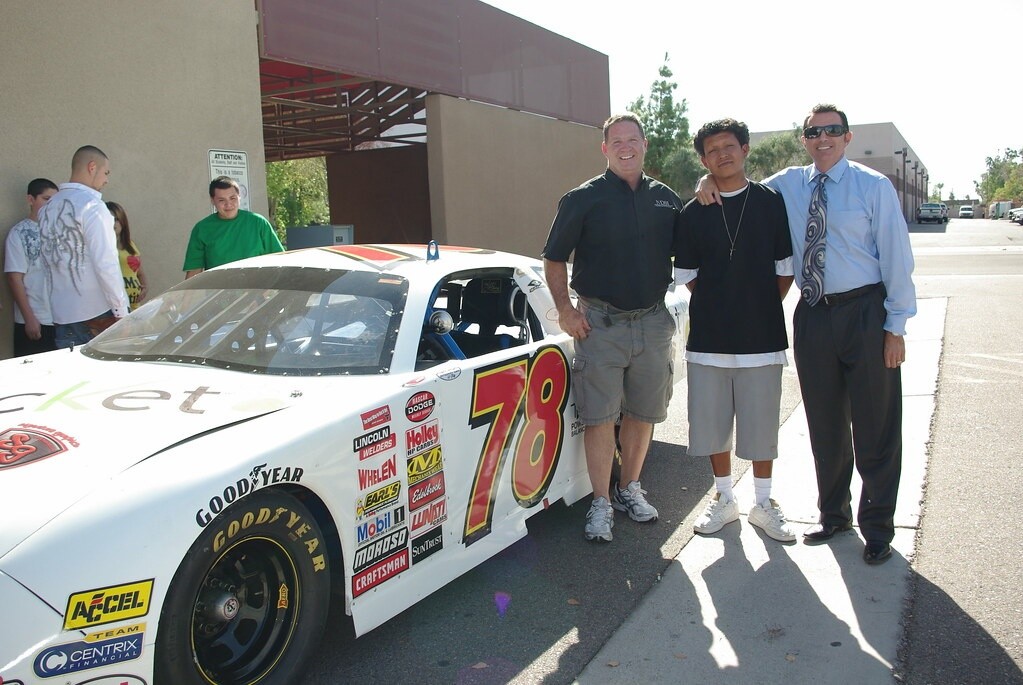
[863,542,893,565]
[802,522,852,540]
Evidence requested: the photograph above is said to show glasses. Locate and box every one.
[803,125,848,139]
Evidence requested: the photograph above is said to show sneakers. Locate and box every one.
[612,480,658,523]
[748,498,797,542]
[692,492,739,534]
[584,496,616,542]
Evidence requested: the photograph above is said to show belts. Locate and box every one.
[814,280,884,307]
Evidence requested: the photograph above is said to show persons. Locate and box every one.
[104,201,149,312]
[692,104,917,565]
[672,118,800,542]
[38,144,131,350]
[540,111,686,543]
[3,178,60,357]
[182,175,286,283]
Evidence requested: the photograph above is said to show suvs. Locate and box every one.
[940,203,950,221]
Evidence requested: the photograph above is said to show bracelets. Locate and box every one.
[892,332,901,337]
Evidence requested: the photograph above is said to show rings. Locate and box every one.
[897,361,902,363]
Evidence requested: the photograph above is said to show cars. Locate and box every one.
[1,238,692,685]
[1008,205,1023,225]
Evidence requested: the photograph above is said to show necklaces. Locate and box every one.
[720,183,751,260]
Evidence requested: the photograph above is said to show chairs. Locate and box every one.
[418,278,532,363]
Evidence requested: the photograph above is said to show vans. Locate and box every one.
[958,205,973,218]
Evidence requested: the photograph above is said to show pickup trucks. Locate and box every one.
[917,203,943,224]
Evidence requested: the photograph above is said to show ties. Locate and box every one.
[801,176,829,308]
